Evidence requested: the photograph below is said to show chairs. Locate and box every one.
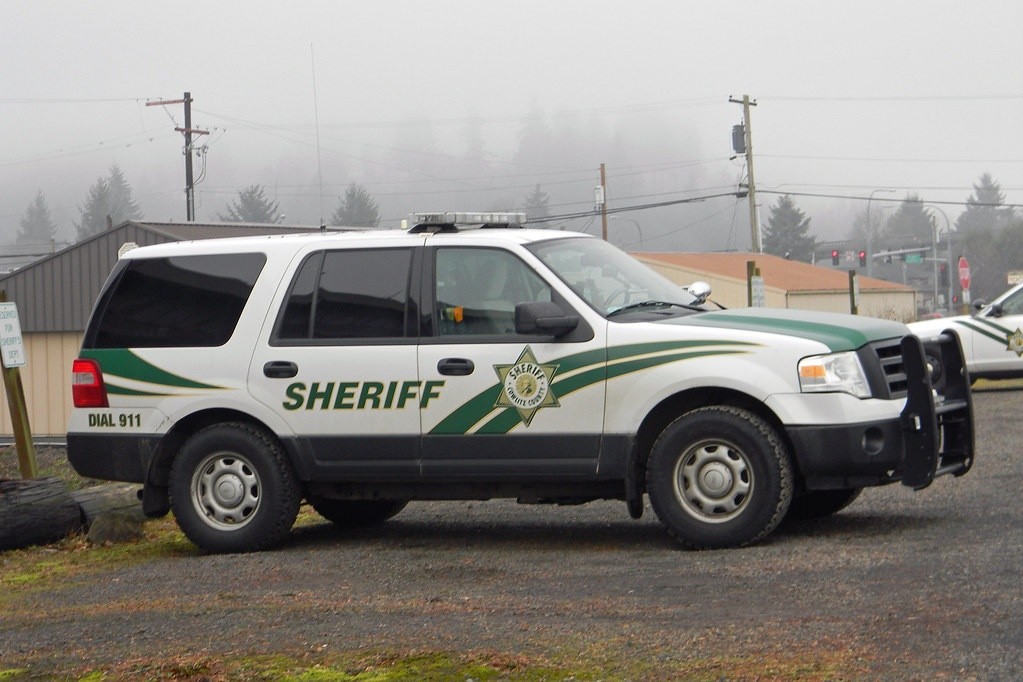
[481,299,517,334]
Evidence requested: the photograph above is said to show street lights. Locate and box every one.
[608,216,645,252]
[865,189,897,277]
[918,204,954,316]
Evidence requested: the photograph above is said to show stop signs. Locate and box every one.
[957,256,971,291]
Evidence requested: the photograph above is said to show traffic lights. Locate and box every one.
[858,250,867,267]
[939,263,949,288]
[898,246,906,262]
[831,249,839,266]
[952,295,958,304]
[919,245,926,259]
[886,248,893,264]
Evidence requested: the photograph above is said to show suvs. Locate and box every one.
[66,210,979,555]
[904,281,1023,388]
